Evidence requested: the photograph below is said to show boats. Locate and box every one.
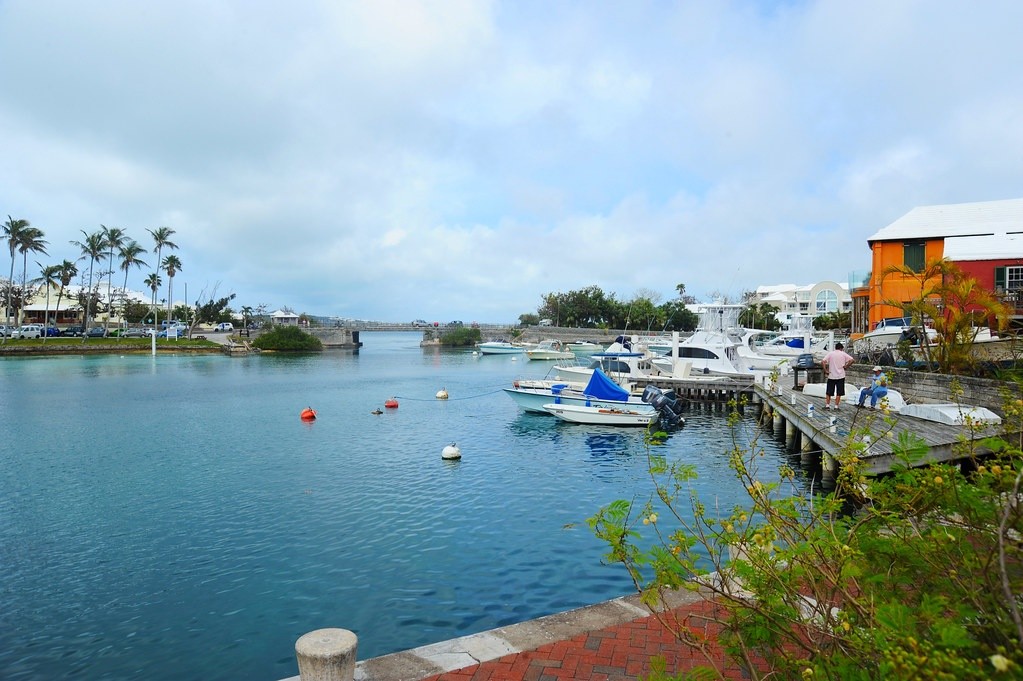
[503,294,1001,427]
[476,334,540,355]
[525,336,576,361]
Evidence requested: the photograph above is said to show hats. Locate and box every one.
[872,366,882,371]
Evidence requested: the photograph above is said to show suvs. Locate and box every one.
[412,319,432,328]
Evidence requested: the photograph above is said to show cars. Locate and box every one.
[143,320,190,340]
[120,327,147,339]
[539,319,553,327]
[0,323,44,338]
[214,322,234,332]
[41,327,61,337]
[61,325,87,338]
[446,320,463,327]
[86,327,110,338]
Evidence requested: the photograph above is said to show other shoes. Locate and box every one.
[822,406,830,411]
[834,407,841,411]
[870,407,875,410]
[856,404,865,408]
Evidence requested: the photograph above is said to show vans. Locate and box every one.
[11,325,41,339]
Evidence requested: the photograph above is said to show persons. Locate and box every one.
[879,343,895,366]
[855,366,888,411]
[822,343,854,411]
